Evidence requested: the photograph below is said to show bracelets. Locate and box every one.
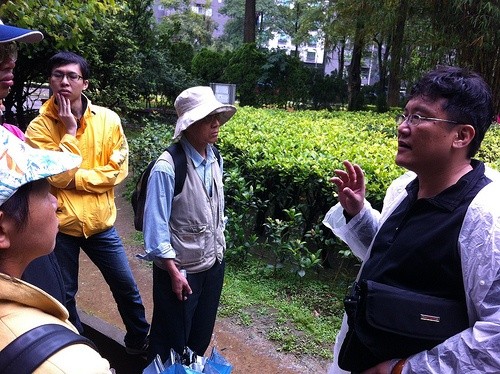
[391,360,405,374]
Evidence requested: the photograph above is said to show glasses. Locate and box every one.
[394,113,464,130]
[0,41,19,67]
[202,113,222,121]
[50,70,84,82]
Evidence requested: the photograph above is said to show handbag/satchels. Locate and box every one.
[338,280,472,372]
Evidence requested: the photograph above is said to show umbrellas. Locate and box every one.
[142,292,234,374]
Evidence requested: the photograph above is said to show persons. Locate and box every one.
[322,65,500,374]
[26,51,150,354]
[0,20,65,305]
[136,86,238,365]
[0,126,116,374]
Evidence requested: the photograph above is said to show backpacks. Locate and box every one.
[131,144,220,231]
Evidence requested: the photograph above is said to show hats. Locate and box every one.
[172,86,237,139]
[0,19,44,44]
[0,125,83,209]
